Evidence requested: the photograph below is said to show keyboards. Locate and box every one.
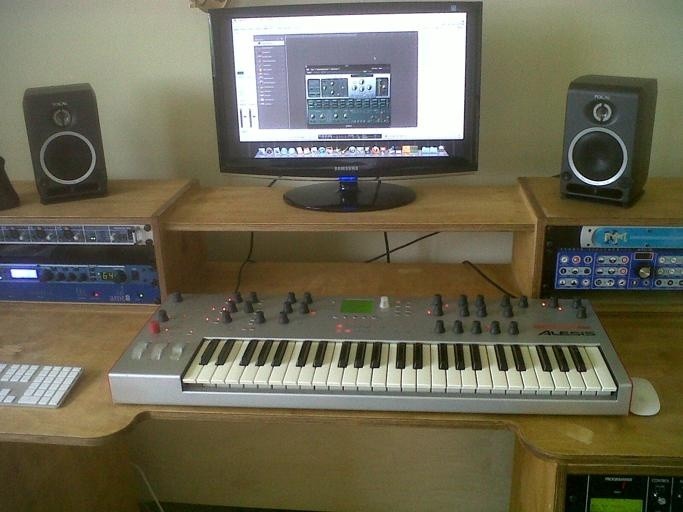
[0,362,85,409]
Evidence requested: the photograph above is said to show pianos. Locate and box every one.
[106,292,633,417]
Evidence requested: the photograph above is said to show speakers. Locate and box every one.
[23,82,108,205]
[559,74,659,209]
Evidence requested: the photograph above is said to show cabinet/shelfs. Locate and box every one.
[0,175,683,511]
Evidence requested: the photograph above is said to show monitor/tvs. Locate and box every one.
[208,0,483,212]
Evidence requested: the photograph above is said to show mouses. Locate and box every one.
[629,377,662,418]
